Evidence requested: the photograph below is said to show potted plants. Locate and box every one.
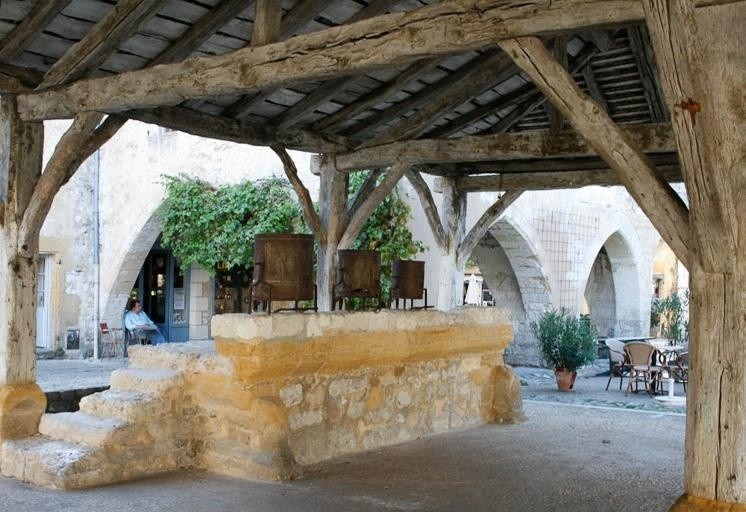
[529,306,600,392]
[651,288,689,382]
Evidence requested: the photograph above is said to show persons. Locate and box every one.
[124,299,166,345]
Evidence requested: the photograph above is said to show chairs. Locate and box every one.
[605,339,688,399]
[100,323,149,362]
